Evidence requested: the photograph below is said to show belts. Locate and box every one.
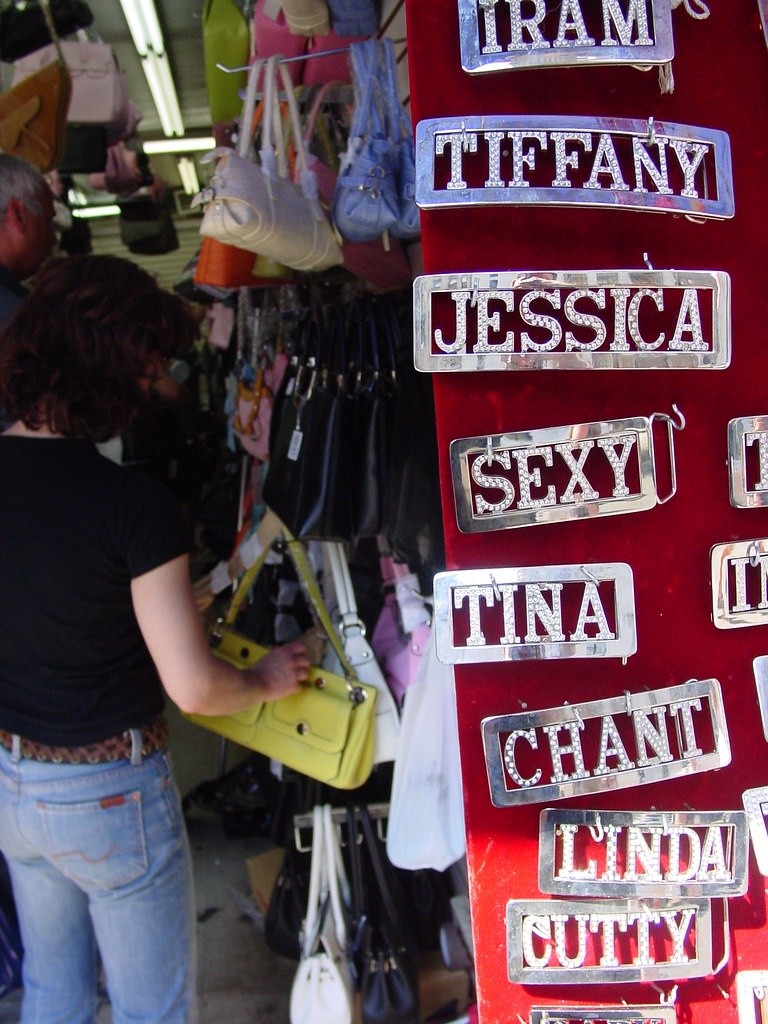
[0,719,170,765]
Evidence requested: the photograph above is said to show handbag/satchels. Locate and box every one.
[185,1,474,1024]
[0,0,179,258]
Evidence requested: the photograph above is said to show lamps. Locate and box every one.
[69,0,219,226]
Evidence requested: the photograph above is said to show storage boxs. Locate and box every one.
[247,839,476,1024]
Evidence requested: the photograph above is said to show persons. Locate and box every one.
[0,252,313,1024]
[0,153,61,431]
